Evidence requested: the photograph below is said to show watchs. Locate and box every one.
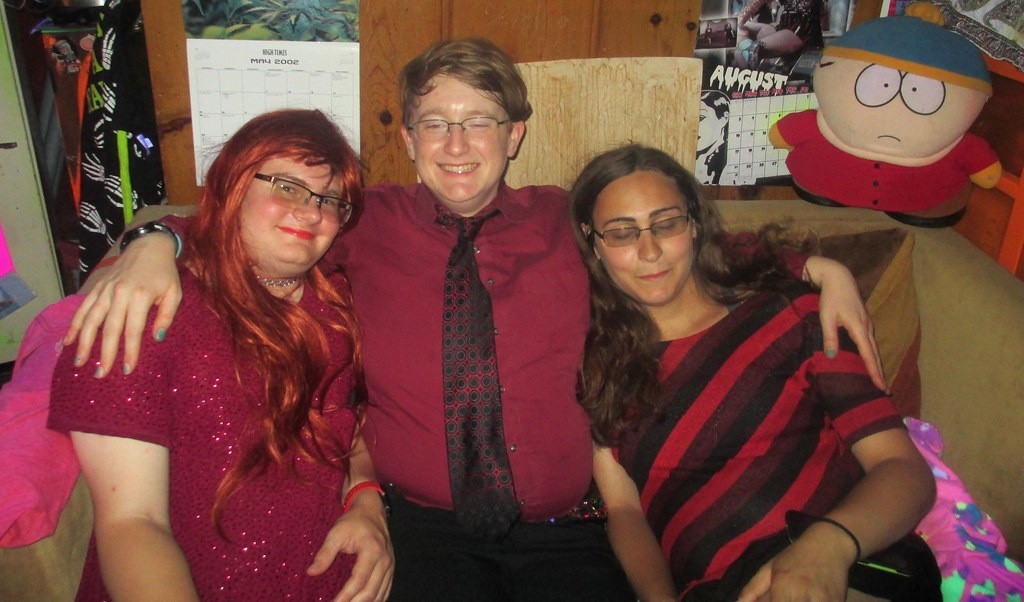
[343,481,394,517]
[118,220,183,258]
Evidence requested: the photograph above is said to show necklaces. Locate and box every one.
[258,276,299,286]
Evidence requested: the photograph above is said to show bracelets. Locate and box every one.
[786,511,860,558]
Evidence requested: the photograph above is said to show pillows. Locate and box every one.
[718,226,920,421]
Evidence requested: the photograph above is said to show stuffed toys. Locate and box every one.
[768,2,1002,228]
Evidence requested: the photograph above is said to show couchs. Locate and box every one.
[0,199,1024,602]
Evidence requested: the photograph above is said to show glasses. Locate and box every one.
[591,208,690,248]
[254,172,353,227]
[406,116,508,143]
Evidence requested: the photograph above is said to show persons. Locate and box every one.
[567,142,943,602]
[704,23,712,44]
[724,22,735,38]
[737,0,824,52]
[65,39,888,602]
[44,108,395,602]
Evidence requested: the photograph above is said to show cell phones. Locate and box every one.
[786,510,913,577]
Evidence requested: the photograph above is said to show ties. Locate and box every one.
[436,209,521,544]
